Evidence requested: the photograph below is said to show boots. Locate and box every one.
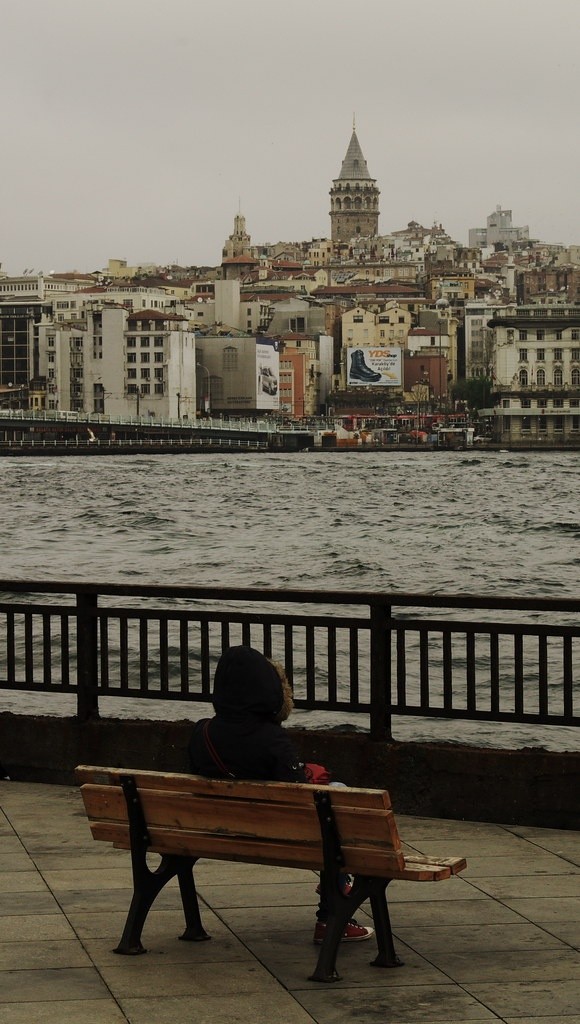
[350,350,381,382]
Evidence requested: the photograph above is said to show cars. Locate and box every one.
[473,434,493,444]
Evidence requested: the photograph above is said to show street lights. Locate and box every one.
[197,361,211,427]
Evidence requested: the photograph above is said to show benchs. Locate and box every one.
[75,764,467,984]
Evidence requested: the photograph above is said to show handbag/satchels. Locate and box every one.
[303,763,330,785]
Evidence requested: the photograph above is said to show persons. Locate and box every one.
[354,432,378,446]
[187,644,374,943]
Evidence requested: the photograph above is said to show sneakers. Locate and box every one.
[315,874,353,895]
[313,918,374,943]
